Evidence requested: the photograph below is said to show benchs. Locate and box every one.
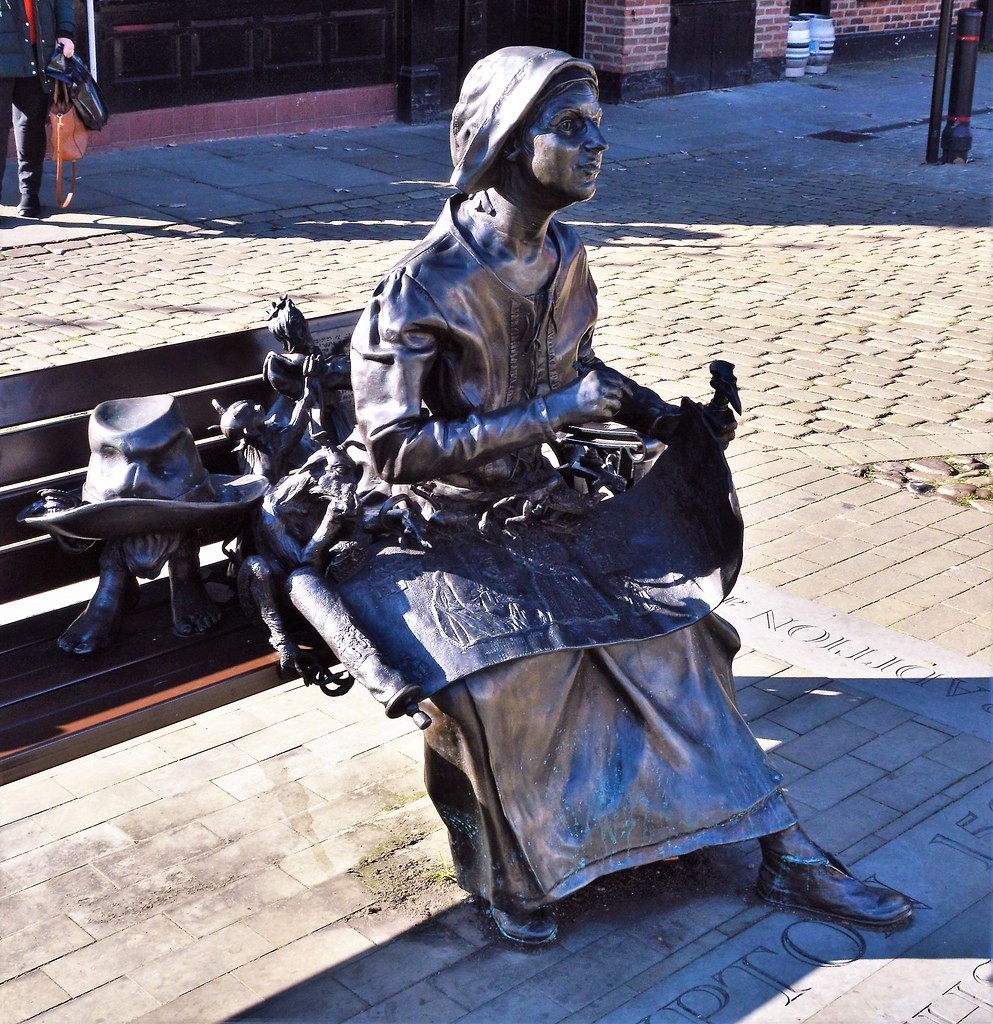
[0,306,366,788]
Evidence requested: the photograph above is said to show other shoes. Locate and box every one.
[18,192,40,217]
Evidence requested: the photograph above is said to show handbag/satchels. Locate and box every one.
[46,77,88,210]
[45,41,109,132]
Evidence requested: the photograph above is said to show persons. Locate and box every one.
[0,0,78,217]
[332,44,914,947]
